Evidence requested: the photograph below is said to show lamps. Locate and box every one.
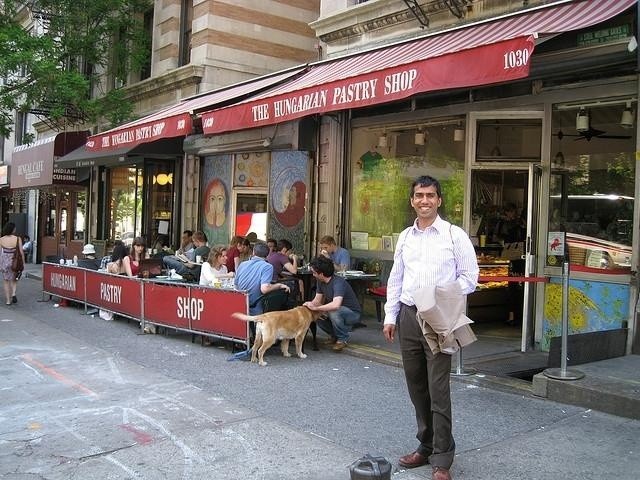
[555,113,565,169]
[489,123,502,163]
[374,119,466,148]
[576,95,638,134]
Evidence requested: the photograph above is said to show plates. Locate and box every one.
[155,275,168,280]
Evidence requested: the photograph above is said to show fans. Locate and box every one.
[552,108,632,143]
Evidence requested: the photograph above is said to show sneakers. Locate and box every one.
[6,303,11,306]
[322,337,335,344]
[332,339,350,351]
[12,294,18,303]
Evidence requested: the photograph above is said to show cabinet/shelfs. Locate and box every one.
[466,244,512,323]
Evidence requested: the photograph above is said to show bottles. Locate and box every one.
[480,225,486,247]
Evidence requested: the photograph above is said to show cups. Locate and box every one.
[196,255,202,264]
[60,258,72,266]
[215,282,222,288]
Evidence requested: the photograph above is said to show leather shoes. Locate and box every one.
[399,451,430,468]
[432,466,452,480]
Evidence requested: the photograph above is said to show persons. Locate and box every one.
[22,234,32,252]
[0,222,25,305]
[59,243,98,306]
[505,208,527,325]
[99,229,352,351]
[205,179,230,227]
[273,181,306,228]
[492,202,518,244]
[302,254,363,352]
[382,175,480,480]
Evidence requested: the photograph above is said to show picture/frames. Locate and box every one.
[380,234,394,253]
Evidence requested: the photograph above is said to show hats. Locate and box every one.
[81,243,96,255]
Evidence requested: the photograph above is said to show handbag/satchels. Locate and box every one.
[12,248,24,272]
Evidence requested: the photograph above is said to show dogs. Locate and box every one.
[231,302,328,367]
